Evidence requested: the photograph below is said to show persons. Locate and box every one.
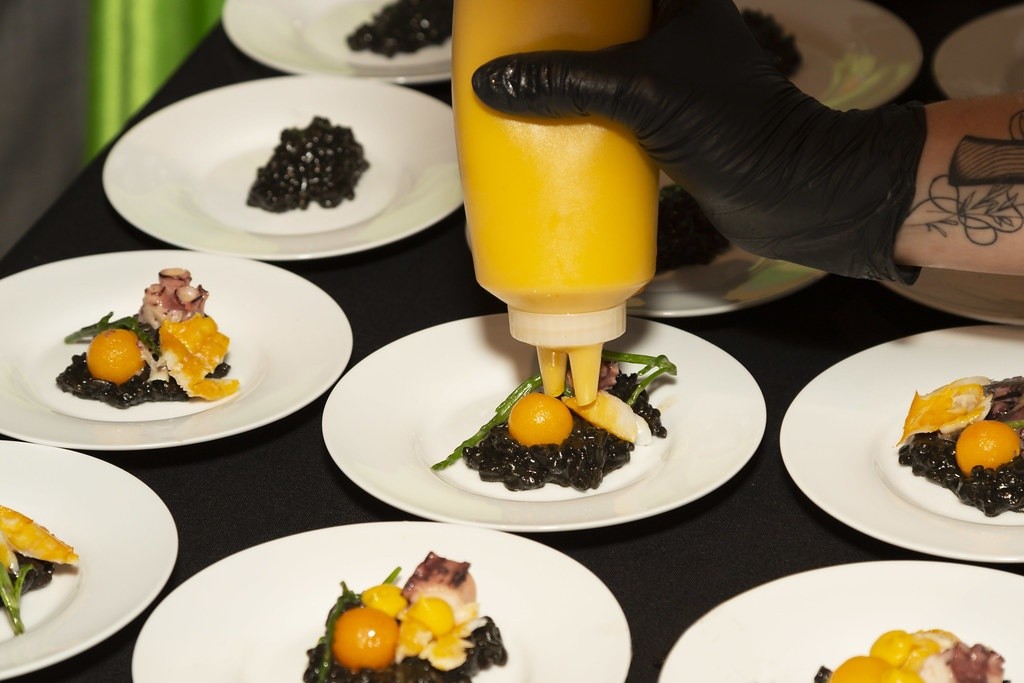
[471,0,1024,278]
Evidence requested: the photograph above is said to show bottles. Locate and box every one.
[452,0,659,408]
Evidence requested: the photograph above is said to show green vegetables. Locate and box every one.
[315,566,401,683]
[0,558,37,635]
[63,312,163,358]
[430,345,677,472]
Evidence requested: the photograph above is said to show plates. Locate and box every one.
[0,440,178,682]
[732,0,924,115]
[221,0,452,82]
[624,166,828,319]
[878,264,1024,327]
[320,311,770,531]
[102,75,463,260]
[655,559,1024,683]
[0,247,353,451]
[780,326,1024,563]
[131,522,633,683]
[933,5,1024,102]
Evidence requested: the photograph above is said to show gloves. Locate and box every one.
[472,0,927,288]
